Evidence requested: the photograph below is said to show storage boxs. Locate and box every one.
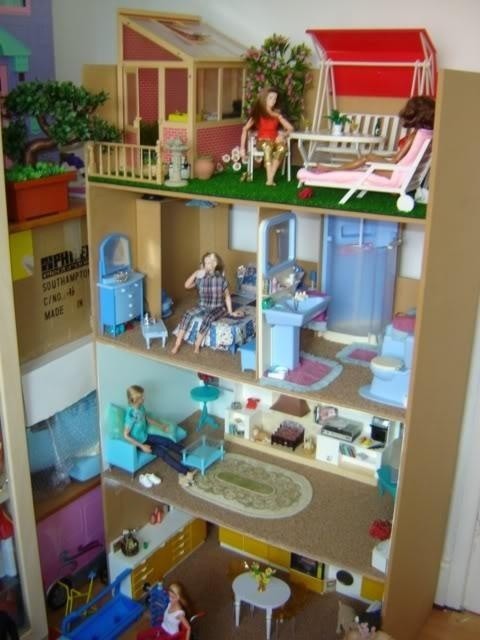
[9,232,107,365]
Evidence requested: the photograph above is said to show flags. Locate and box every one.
[306,94,437,179]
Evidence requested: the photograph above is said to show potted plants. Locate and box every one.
[0,79,130,224]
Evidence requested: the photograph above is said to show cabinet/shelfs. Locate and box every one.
[61,60,480,640]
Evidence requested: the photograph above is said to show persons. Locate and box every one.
[123,383,199,480]
[237,89,295,187]
[170,251,245,356]
[136,583,195,640]
